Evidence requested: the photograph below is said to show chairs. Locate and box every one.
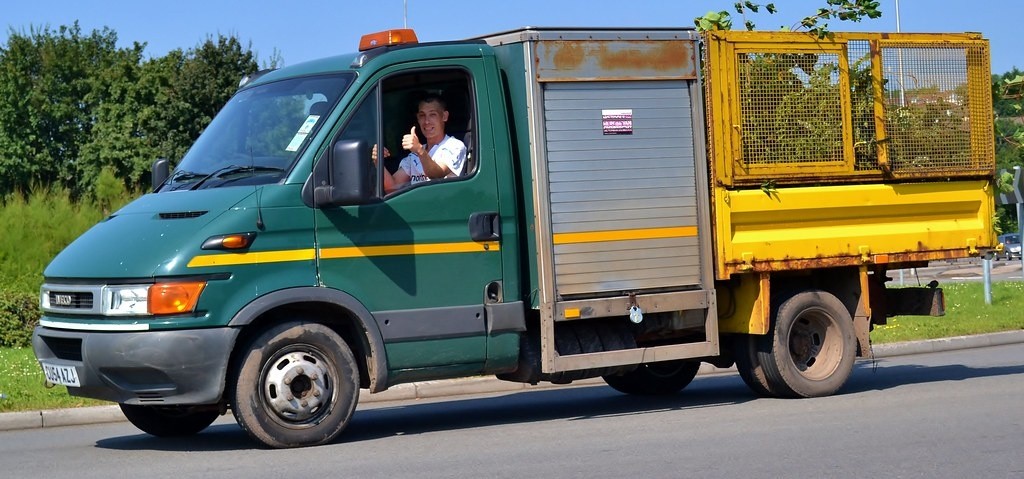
[445,110,473,176]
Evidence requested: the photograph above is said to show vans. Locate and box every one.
[995,233,1022,261]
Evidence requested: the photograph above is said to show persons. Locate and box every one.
[371,84,468,194]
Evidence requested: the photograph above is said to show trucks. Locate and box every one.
[29,26,1004,444]
